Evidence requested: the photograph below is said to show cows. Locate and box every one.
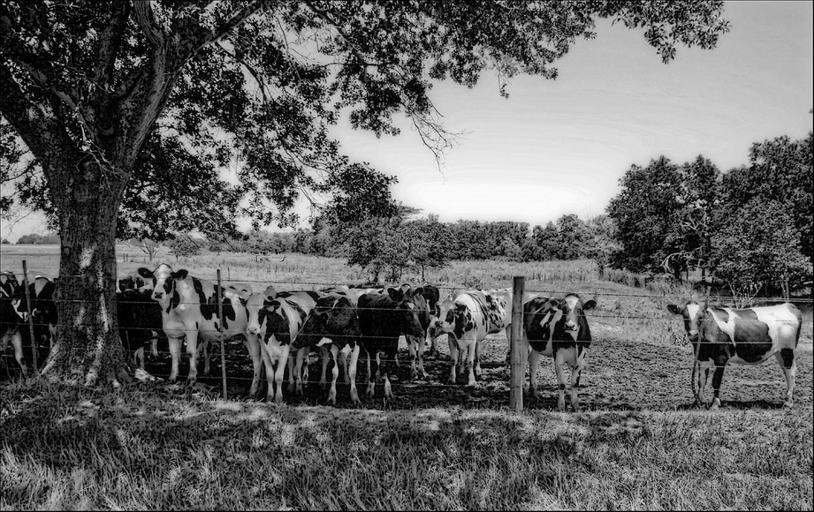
[426,285,517,389]
[0,261,442,406]
[521,291,599,412]
[669,300,804,409]
